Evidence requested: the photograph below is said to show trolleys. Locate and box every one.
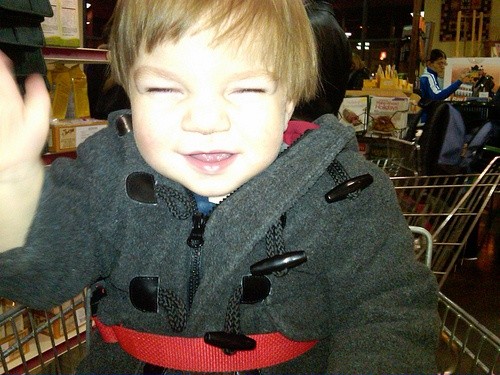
[369,144,500,289]
[0,221,500,375]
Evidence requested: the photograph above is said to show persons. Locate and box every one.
[0,0,441,375]
[345,49,370,90]
[420,49,472,124]
[291,6,351,127]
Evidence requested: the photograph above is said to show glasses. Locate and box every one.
[430,62,448,67]
